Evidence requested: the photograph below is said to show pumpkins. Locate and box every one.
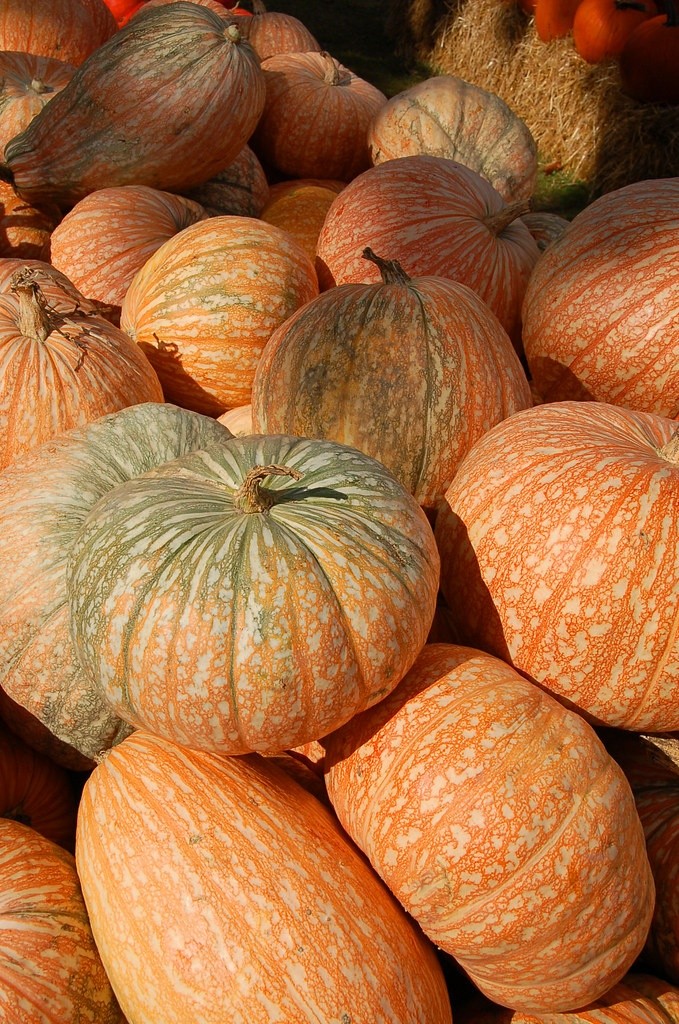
[0,0,679,1024]
